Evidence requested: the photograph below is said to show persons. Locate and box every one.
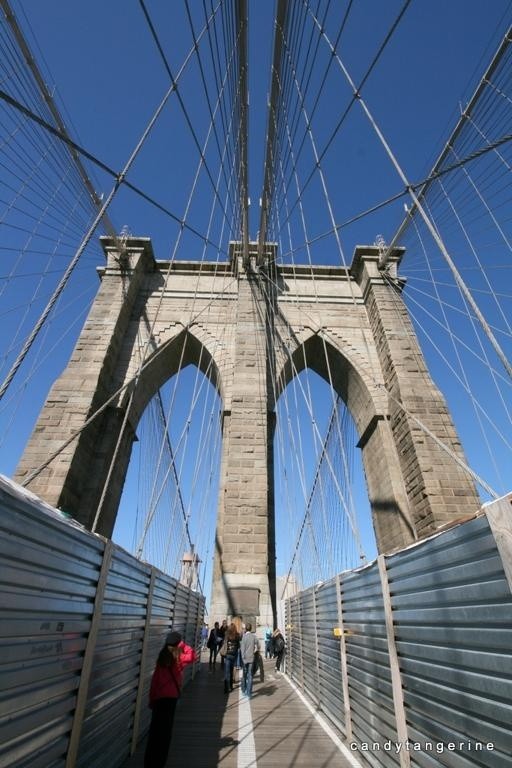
[142,630,198,768]
[203,620,285,698]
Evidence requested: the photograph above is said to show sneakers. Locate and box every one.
[242,690,253,699]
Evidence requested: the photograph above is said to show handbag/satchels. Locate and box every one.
[219,638,228,657]
